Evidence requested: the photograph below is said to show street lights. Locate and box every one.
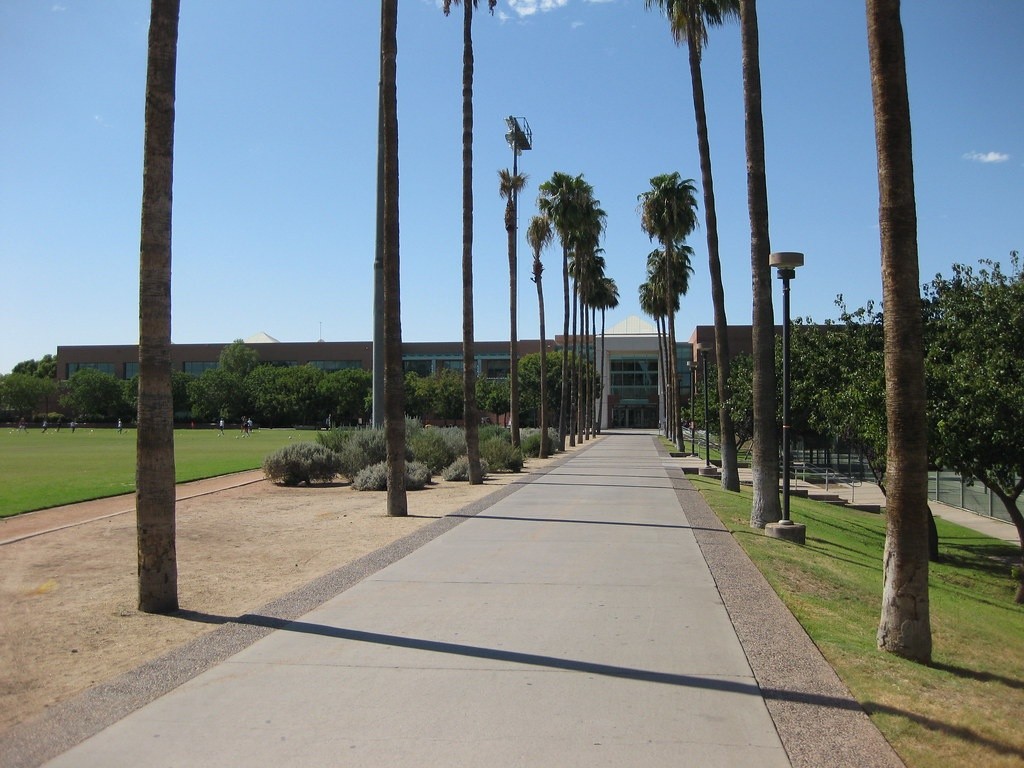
[695,341,713,468]
[686,360,699,458]
[769,251,806,528]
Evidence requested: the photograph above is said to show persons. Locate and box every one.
[19,418,124,434]
[219,414,335,437]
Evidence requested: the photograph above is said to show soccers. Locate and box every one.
[242,435,245,438]
[218,434,221,437]
[288,435,292,439]
[236,436,239,439]
